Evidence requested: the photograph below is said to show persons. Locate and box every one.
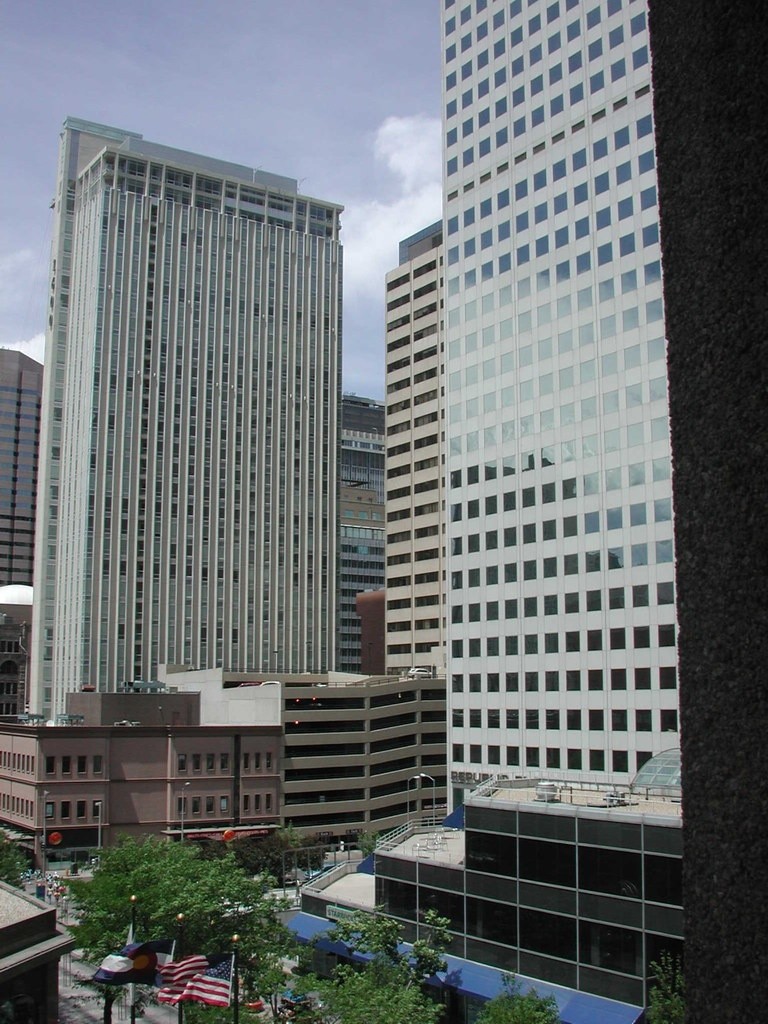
[20,867,67,905]
[278,996,287,1015]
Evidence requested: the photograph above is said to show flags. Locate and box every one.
[157,953,233,1006]
[93,940,173,986]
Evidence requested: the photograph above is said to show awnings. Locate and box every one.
[441,803,464,829]
[356,854,374,875]
[283,912,644,1024]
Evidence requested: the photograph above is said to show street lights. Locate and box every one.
[94,801,103,864]
[406,776,420,829]
[420,773,435,826]
[43,789,50,878]
[181,782,190,842]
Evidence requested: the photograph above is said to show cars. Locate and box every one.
[238,983,342,1024]
[237,680,281,689]
[407,667,434,679]
[277,862,337,889]
